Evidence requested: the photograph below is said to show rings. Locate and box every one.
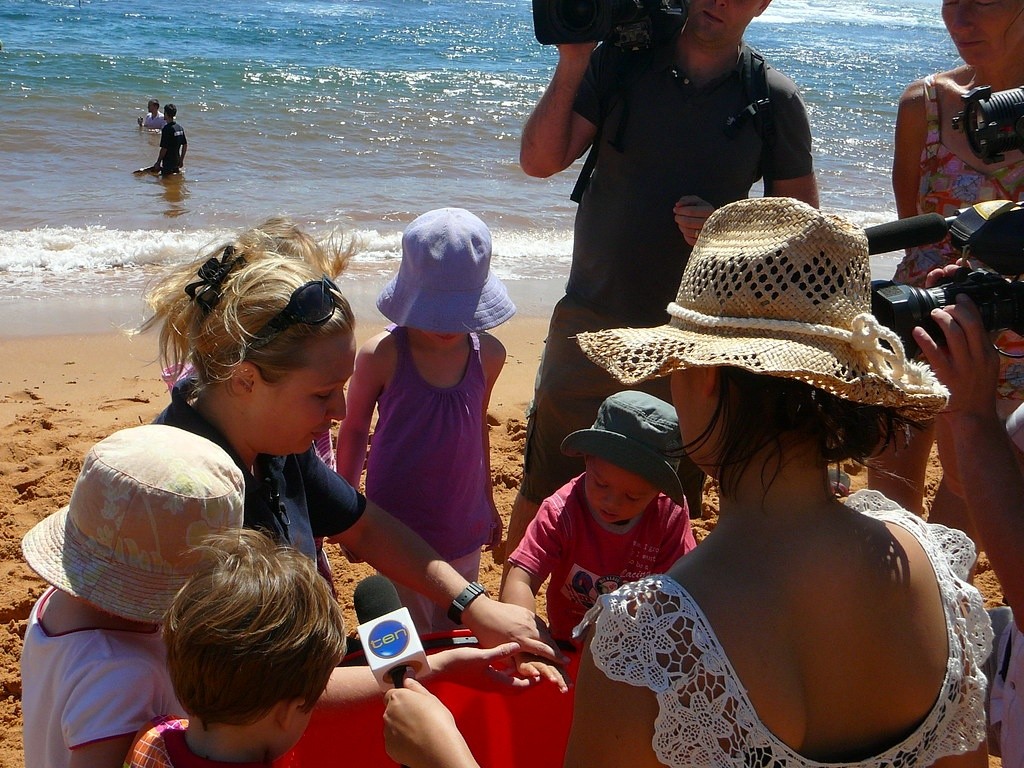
[695,230,698,238]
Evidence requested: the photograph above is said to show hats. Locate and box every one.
[577,197,950,422]
[21,425,246,622]
[561,391,687,507]
[376,207,517,333]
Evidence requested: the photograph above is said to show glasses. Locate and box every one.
[228,274,342,363]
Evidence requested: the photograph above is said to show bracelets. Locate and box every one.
[445,581,489,626]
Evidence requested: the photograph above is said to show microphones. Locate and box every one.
[353,575,433,693]
[865,213,960,255]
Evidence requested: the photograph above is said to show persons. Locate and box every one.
[21,0,1023,768]
